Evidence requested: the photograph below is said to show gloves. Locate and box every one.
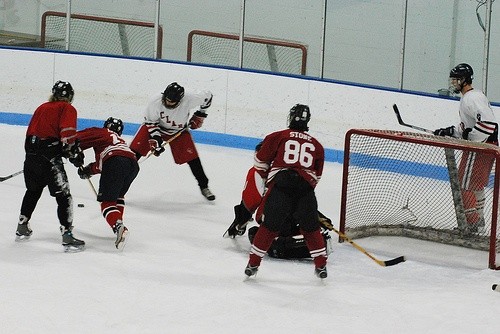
[188,112,208,129]
[67,146,85,168]
[78,162,94,179]
[228,206,251,236]
[433,126,454,137]
[148,135,165,157]
[462,127,472,139]
[317,211,332,232]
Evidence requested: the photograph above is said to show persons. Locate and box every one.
[15,81,86,253]
[434,63,499,232]
[229,141,333,262]
[63,117,140,252]
[130,82,216,201]
[244,104,328,285]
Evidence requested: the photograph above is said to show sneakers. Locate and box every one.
[15,216,32,241]
[113,222,129,249]
[201,187,215,204]
[60,226,86,252]
[243,261,259,281]
[455,216,486,235]
[314,266,328,284]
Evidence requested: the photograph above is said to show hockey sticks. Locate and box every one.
[133,123,191,164]
[79,164,98,197]
[316,215,407,267]
[0,170,24,182]
[393,104,435,134]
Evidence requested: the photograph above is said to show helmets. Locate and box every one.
[287,103,311,132]
[52,81,74,102]
[104,118,123,135]
[448,63,473,94]
[162,82,184,108]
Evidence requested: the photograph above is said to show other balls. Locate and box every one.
[78,204,84,207]
